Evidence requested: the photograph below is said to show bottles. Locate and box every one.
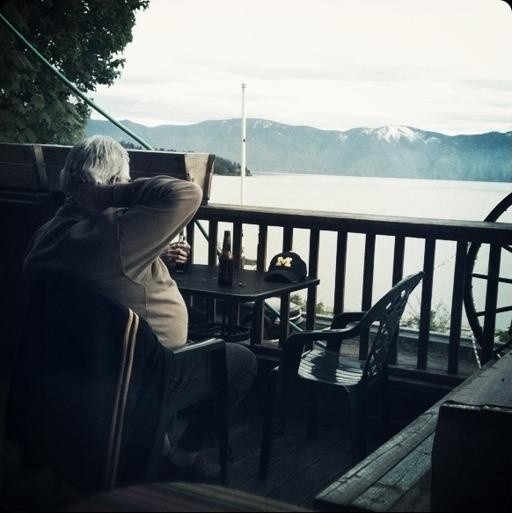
[218,230,235,287]
[174,228,187,274]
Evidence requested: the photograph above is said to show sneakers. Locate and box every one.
[162,455,225,480]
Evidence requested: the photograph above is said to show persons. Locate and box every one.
[21,136,260,485]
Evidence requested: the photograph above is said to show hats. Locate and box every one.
[265,252,307,283]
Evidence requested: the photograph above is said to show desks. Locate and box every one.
[171,265,319,461]
